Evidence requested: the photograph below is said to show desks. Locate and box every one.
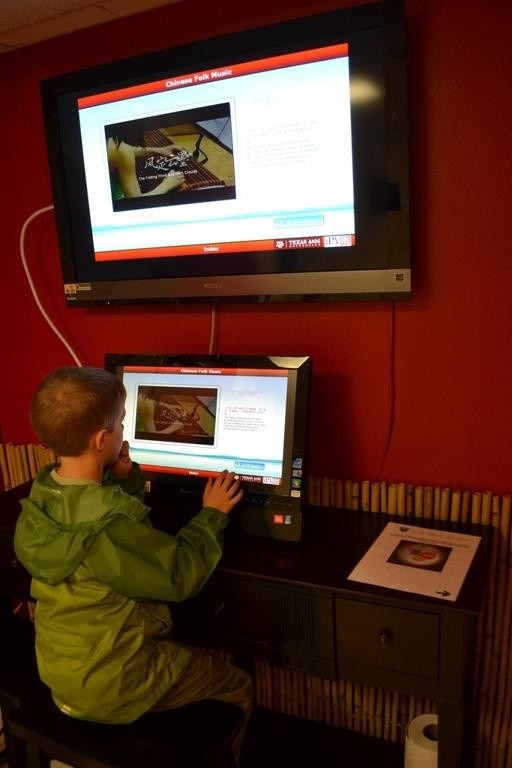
[2,472,499,765]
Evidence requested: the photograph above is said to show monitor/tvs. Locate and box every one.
[39,0,411,305]
[103,351,311,541]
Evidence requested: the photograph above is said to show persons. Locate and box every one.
[11,365,255,766]
[136,385,185,436]
[107,126,186,201]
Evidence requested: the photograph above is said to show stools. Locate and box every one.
[2,669,250,766]
